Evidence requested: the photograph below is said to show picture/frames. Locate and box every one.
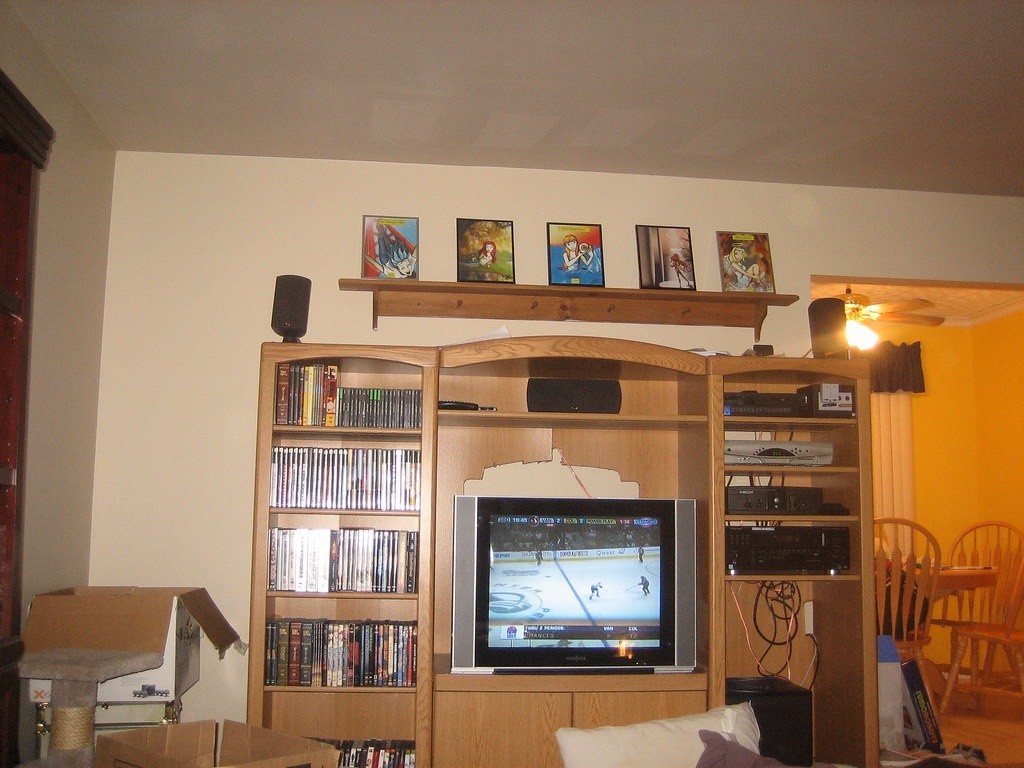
[635,225,696,291]
[716,231,777,294]
[546,222,605,288]
[455,218,515,284]
[361,214,419,282]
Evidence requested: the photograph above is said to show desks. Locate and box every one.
[897,561,998,707]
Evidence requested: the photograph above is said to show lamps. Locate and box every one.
[842,318,881,351]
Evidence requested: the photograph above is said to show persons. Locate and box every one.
[875,557,928,637]
[535,551,543,567]
[638,546,643,562]
[493,523,660,552]
[638,576,650,595]
[589,582,602,600]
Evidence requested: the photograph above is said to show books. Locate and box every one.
[267,527,419,593]
[303,737,415,768]
[269,445,420,511]
[335,386,422,429]
[264,619,417,688]
[277,363,338,426]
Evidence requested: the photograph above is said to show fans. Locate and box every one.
[835,283,944,326]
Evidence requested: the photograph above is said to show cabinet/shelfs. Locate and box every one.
[245,337,882,768]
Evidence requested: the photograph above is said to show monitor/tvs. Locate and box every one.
[451,495,696,676]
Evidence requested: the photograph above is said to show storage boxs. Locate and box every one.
[900,659,947,752]
[24,583,239,706]
[95,717,340,767]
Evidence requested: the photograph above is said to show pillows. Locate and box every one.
[555,702,762,768]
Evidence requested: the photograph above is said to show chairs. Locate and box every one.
[872,517,1024,714]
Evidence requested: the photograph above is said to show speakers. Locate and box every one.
[810,297,849,359]
[271,275,311,343]
[526,378,622,413]
